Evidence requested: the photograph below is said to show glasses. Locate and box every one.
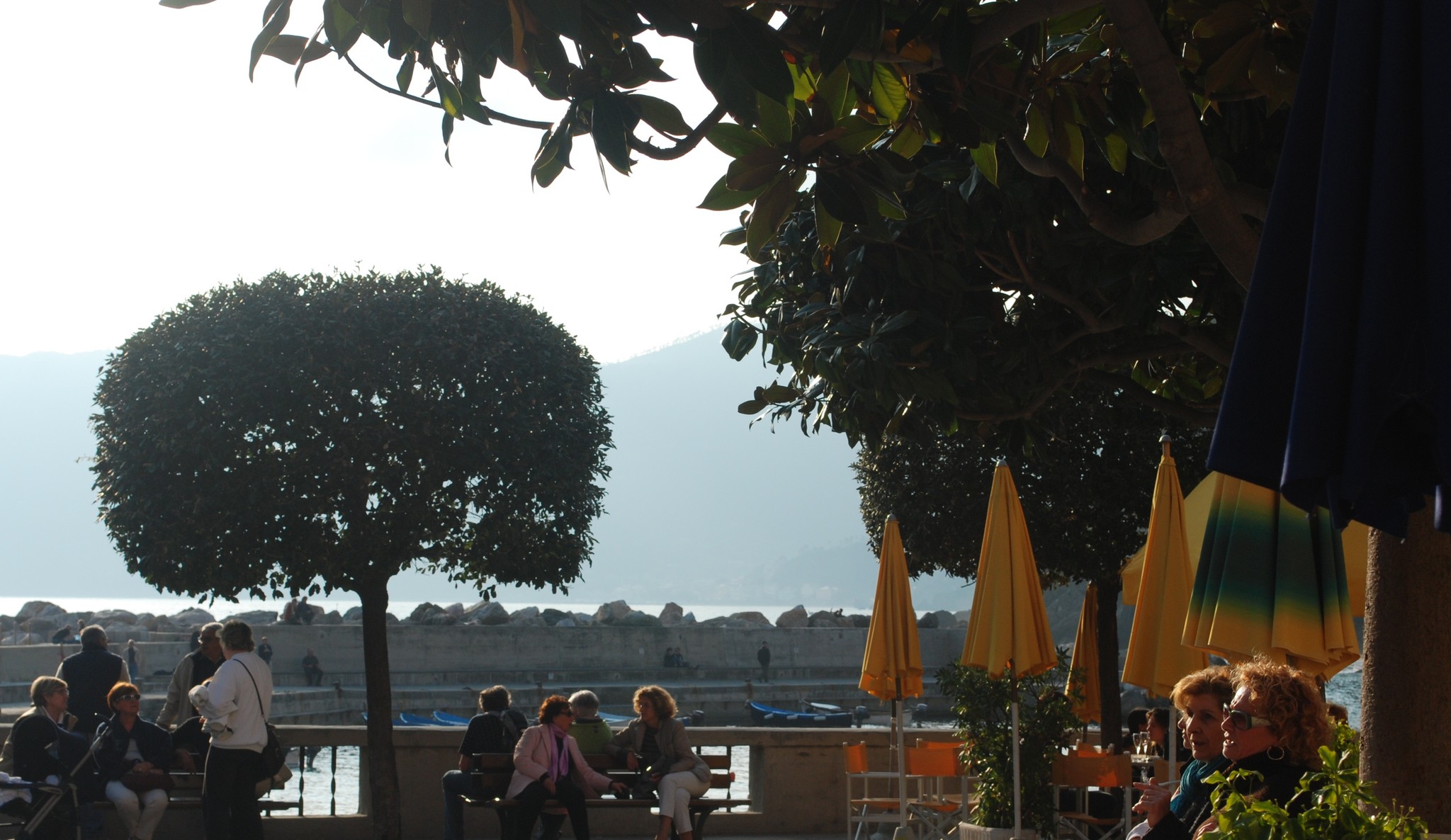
[51,689,69,696]
[198,636,220,644]
[1221,704,1280,731]
[115,694,141,702]
[557,711,573,717]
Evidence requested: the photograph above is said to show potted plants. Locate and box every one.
[930,644,1092,840]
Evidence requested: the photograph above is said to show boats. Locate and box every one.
[594,709,706,727]
[745,698,872,729]
[433,711,471,726]
[398,713,454,727]
[359,709,405,726]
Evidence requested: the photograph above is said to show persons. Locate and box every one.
[757,641,770,683]
[152,621,227,732]
[1100,647,1360,840]
[440,684,712,840]
[93,682,174,840]
[0,676,95,840]
[55,624,132,746]
[188,619,273,840]
[122,638,140,685]
[258,596,324,687]
[664,647,690,667]
[51,618,87,644]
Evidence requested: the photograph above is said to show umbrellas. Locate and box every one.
[1117,425,1369,805]
[858,515,925,840]
[1202,0,1451,552]
[961,455,1059,840]
[1064,577,1101,744]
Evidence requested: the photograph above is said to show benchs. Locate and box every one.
[90,734,297,813]
[454,746,754,840]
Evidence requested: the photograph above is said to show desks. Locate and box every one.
[1130,752,1163,768]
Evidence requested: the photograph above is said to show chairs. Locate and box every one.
[837,734,1137,840]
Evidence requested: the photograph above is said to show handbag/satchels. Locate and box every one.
[119,758,175,794]
[261,728,284,779]
